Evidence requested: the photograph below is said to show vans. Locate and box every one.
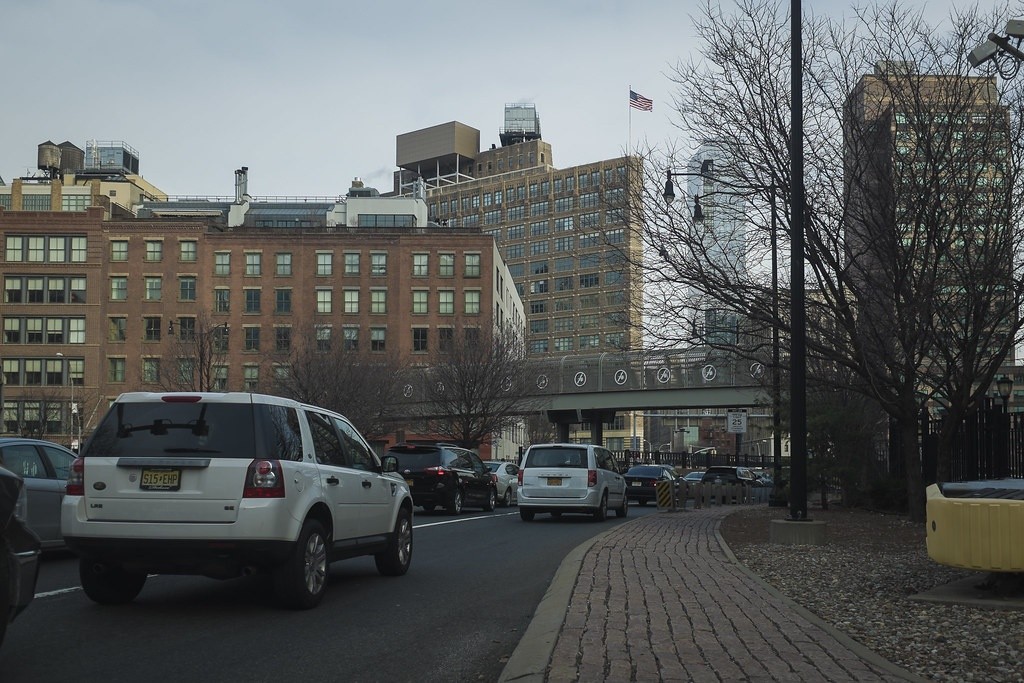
[517,444,629,520]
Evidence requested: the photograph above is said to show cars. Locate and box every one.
[701,465,764,505]
[472,461,520,507]
[621,464,687,505]
[1,436,80,551]
[683,470,706,488]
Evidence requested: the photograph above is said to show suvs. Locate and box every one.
[387,441,498,516]
[59,389,415,608]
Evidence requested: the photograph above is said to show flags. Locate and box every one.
[630,91,653,112]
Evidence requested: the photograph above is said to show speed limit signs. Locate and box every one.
[727,411,747,434]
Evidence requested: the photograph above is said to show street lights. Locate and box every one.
[663,168,788,508]
[169,322,228,392]
[996,375,1012,478]
[56,353,74,442]
